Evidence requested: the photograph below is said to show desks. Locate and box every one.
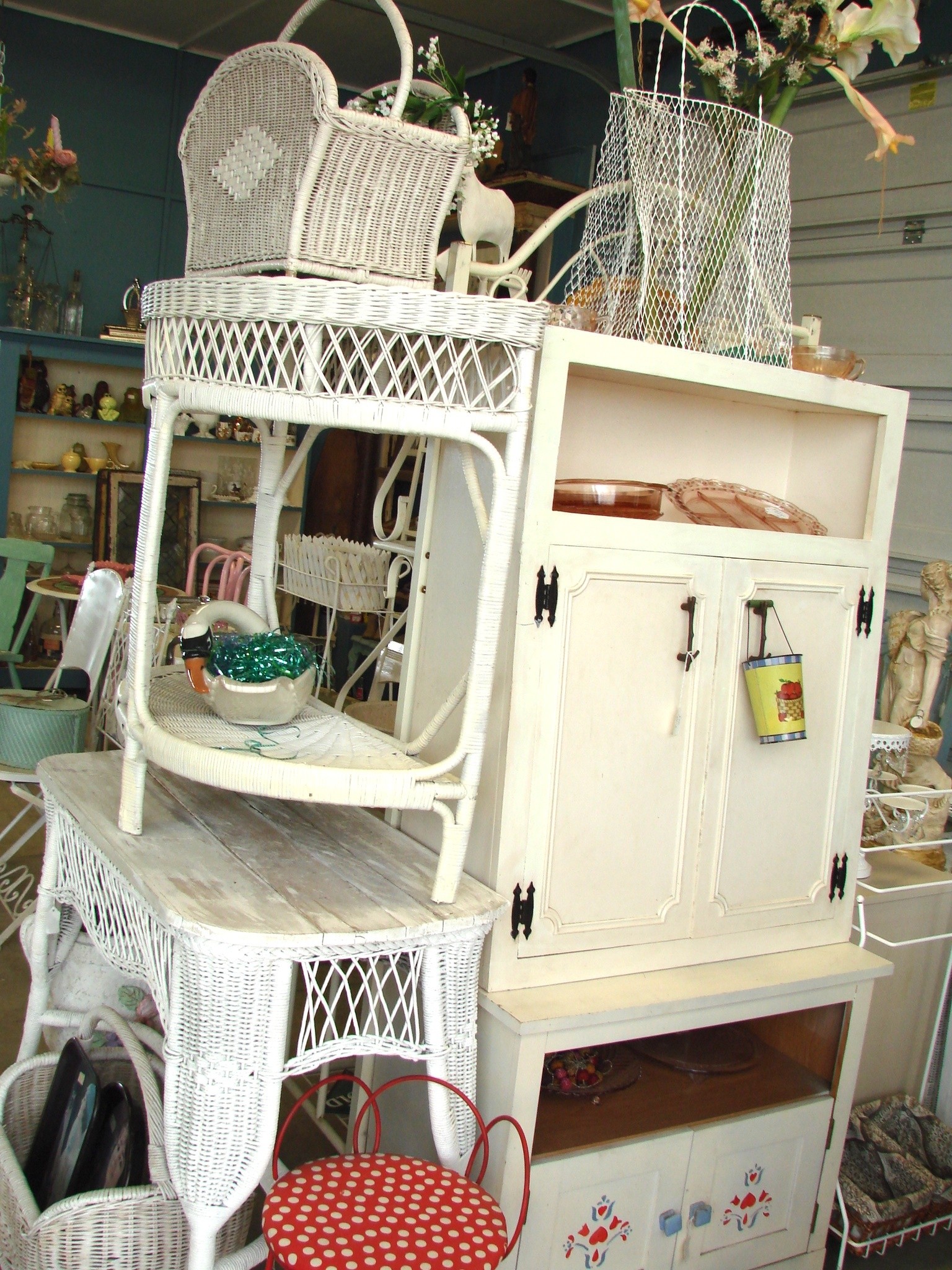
[26,576,187,689]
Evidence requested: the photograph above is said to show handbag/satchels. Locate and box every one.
[558,1,793,369]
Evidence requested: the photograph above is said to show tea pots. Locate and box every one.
[61,448,80,473]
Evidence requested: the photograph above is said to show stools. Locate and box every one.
[260,1075,531,1269]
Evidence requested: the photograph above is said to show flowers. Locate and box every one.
[345,34,502,217]
[624,0,922,338]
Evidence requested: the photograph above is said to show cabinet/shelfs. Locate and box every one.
[0,277,952,1270]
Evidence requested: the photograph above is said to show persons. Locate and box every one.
[880,559,952,790]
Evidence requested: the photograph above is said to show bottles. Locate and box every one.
[38,600,63,662]
[32,284,59,334]
[59,493,91,540]
[72,442,88,472]
[199,536,227,563]
[7,511,26,539]
[12,593,40,663]
[26,506,57,543]
[59,270,83,336]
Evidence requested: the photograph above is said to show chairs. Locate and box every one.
[186,542,243,601]
[0,538,57,689]
[44,569,125,701]
[220,550,253,608]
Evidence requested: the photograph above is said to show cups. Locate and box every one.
[215,422,297,446]
[200,481,217,501]
[228,480,240,493]
[70,514,88,543]
[84,457,114,475]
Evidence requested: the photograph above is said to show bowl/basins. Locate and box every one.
[552,478,666,521]
[790,345,865,381]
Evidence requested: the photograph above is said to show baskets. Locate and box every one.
[1,1004,255,1270]
[179,1,473,289]
[828,1093,952,1256]
[283,534,391,610]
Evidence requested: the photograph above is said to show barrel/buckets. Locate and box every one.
[741,654,807,745]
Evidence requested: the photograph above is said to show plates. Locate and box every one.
[542,1045,641,1096]
[24,1038,138,1212]
[212,495,241,502]
[638,1028,762,1072]
[664,478,829,537]
[31,462,59,471]
